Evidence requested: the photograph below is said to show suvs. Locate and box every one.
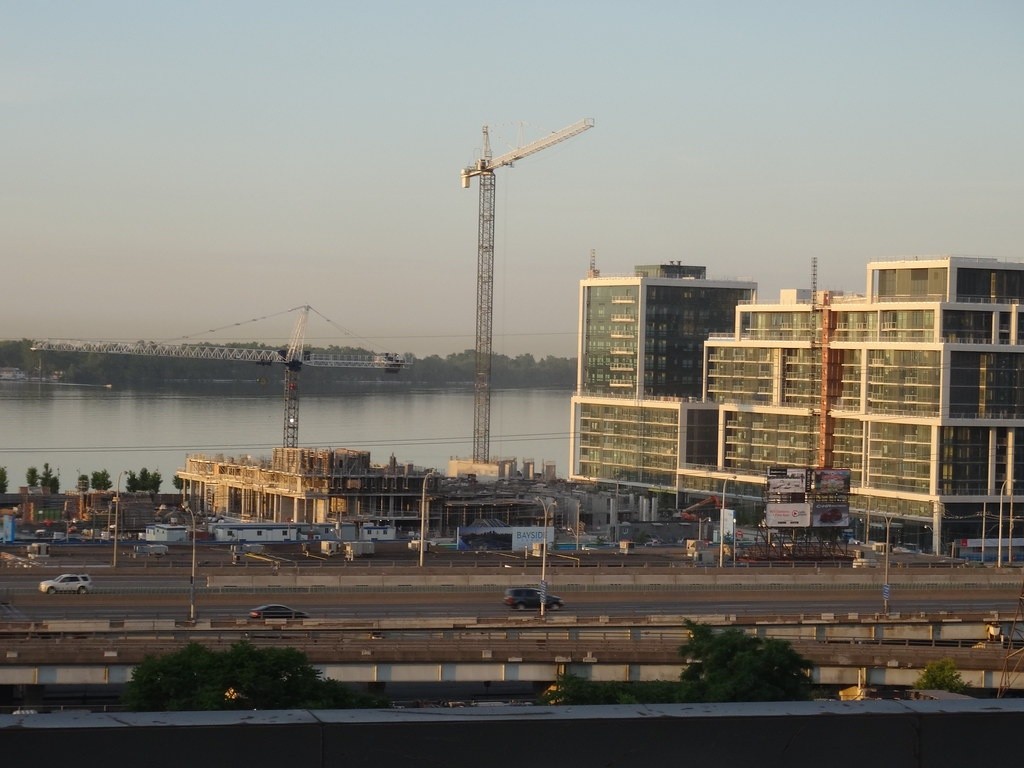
[504,587,564,612]
[39,574,93,597]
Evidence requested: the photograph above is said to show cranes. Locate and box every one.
[457,117,603,466]
[30,302,411,449]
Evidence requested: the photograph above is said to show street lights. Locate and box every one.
[882,511,900,614]
[114,470,128,567]
[732,518,737,565]
[698,518,711,540]
[998,478,1017,570]
[535,496,558,616]
[181,501,197,621]
[719,475,737,566]
[863,493,876,547]
[419,472,436,568]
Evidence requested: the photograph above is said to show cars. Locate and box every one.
[249,604,310,620]
